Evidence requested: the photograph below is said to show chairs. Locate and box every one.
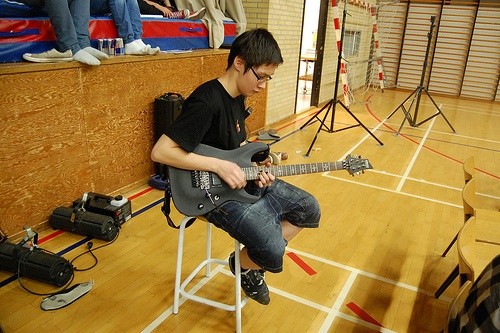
[435,156,500,333]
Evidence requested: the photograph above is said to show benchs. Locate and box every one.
[299,74,313,81]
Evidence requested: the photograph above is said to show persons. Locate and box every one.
[151,28,320,305]
[45,0,108,66]
[137,0,206,21]
[455,254,500,333]
[90,0,159,54]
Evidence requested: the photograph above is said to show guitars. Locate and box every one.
[167,142,374,217]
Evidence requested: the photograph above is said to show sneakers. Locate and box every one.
[188,7,206,20]
[229,250,270,305]
[22,47,74,62]
[168,9,190,19]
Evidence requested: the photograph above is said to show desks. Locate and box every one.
[300,57,315,94]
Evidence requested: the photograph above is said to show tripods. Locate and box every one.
[387,16,455,136]
[300,0,384,157]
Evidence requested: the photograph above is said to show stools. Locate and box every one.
[173,216,265,333]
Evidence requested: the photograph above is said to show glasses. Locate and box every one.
[249,66,272,82]
